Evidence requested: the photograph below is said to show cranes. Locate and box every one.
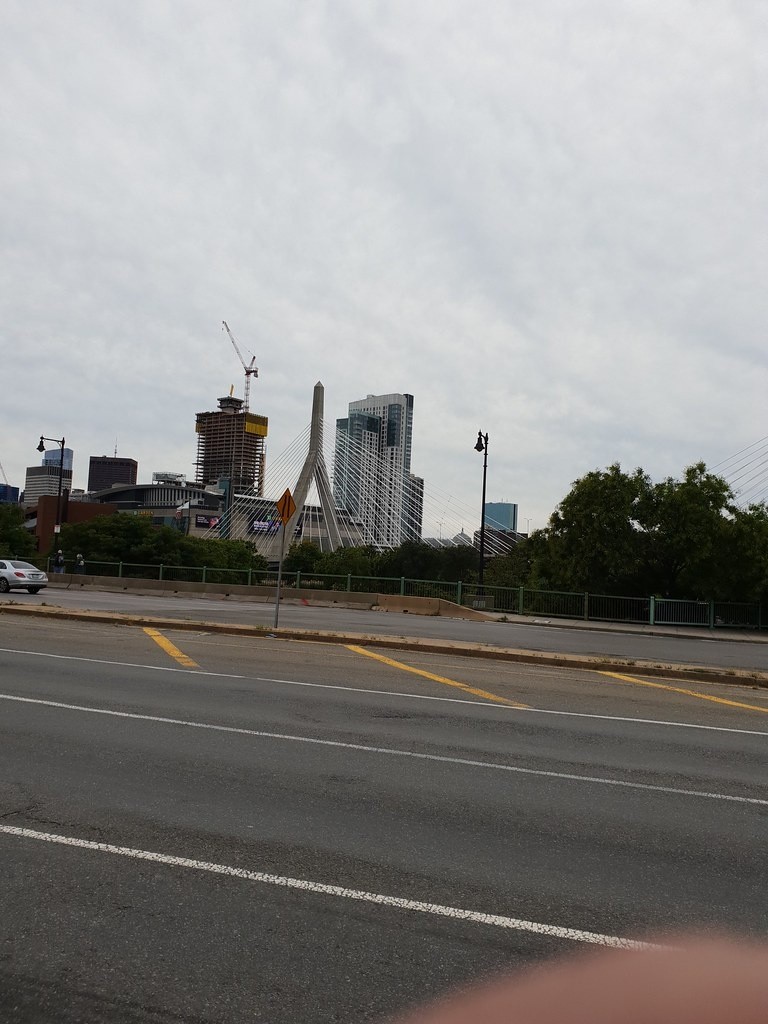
[436,495,452,542]
[222,321,258,412]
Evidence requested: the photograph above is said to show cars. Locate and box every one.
[0,560,48,594]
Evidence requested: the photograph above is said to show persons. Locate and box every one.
[76,554,86,574]
[52,550,64,573]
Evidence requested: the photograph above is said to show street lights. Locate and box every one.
[36,437,65,559]
[474,429,489,596]
[524,518,532,533]
